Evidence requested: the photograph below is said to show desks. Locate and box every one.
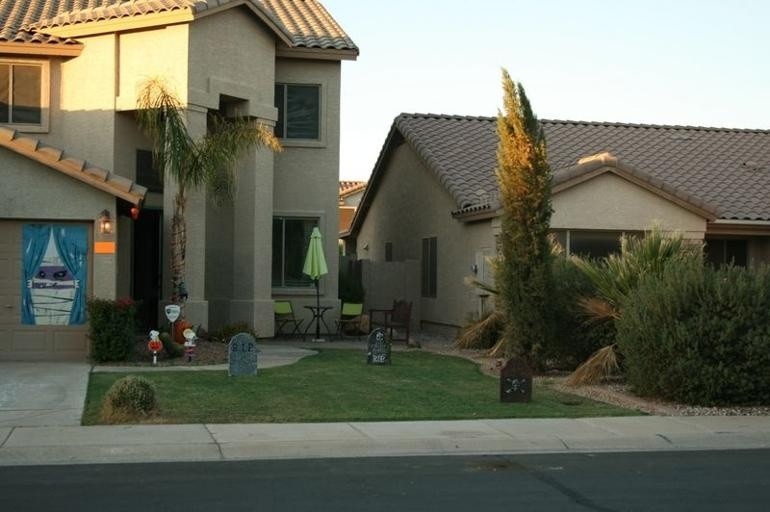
[301,305,335,342]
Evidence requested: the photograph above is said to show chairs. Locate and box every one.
[272,299,304,338]
[332,298,413,345]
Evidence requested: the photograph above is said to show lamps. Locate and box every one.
[98,210,113,234]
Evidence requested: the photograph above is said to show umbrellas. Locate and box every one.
[302,226,329,338]
